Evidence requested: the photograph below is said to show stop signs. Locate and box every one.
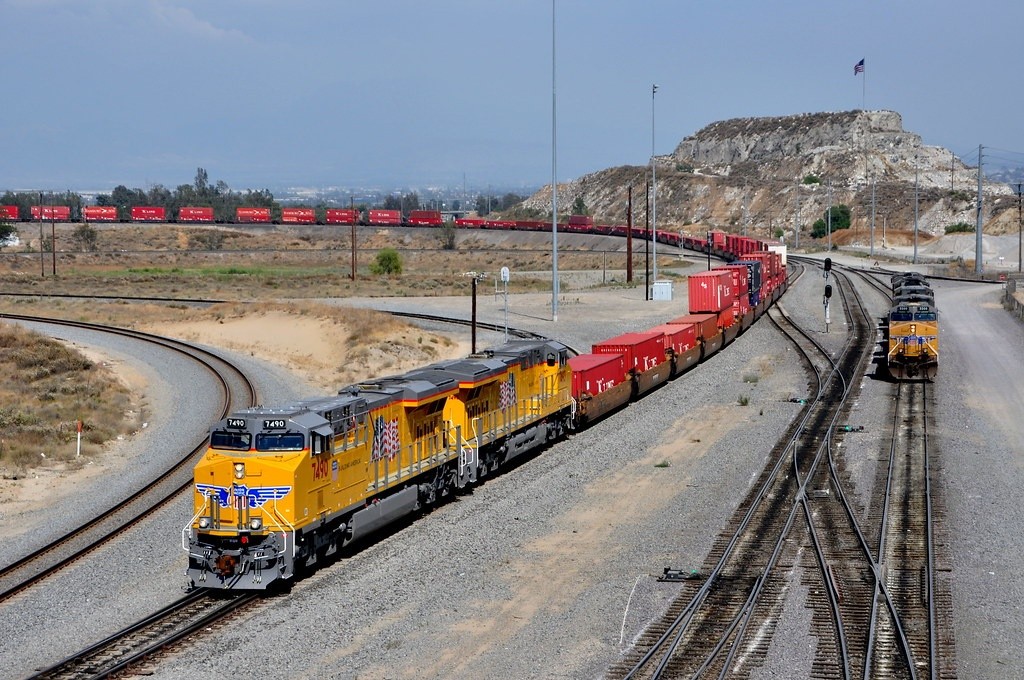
[1000,275,1006,280]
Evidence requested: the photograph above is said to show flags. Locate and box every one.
[371,415,400,463]
[498,372,517,413]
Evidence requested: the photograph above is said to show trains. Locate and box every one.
[887,272,940,381]
[0,205,790,595]
